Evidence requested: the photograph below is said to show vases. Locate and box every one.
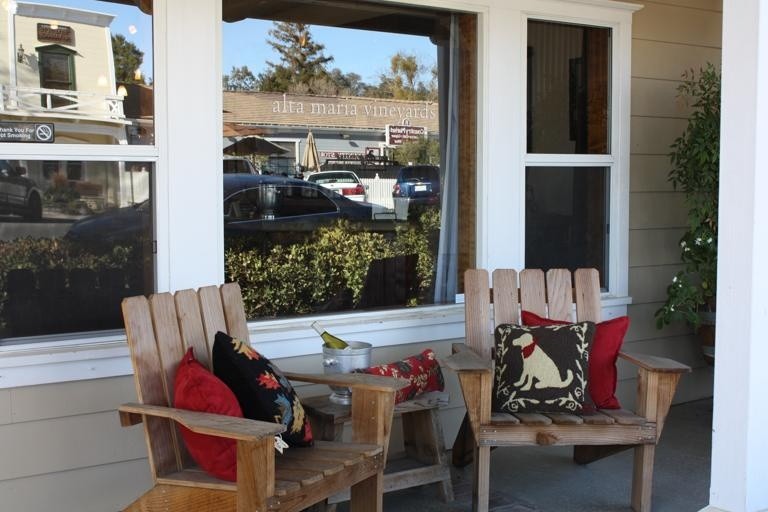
[700,326,713,367]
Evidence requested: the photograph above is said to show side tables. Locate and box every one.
[299,390,455,512]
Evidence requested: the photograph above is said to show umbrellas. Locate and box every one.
[224,135,290,170]
[299,127,324,199]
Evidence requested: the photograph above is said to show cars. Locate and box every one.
[393,165,441,212]
[306,171,368,203]
[61,175,397,257]
[68,183,104,212]
[0,159,42,220]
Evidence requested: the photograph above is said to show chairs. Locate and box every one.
[115,283,411,512]
[442,267,691,512]
[356,253,418,307]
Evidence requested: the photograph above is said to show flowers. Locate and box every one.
[653,222,716,329]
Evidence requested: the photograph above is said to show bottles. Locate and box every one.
[311,320,354,350]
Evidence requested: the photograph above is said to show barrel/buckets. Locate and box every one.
[322,342,373,406]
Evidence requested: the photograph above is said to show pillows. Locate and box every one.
[171,330,314,485]
[492,310,630,415]
[350,348,444,416]
